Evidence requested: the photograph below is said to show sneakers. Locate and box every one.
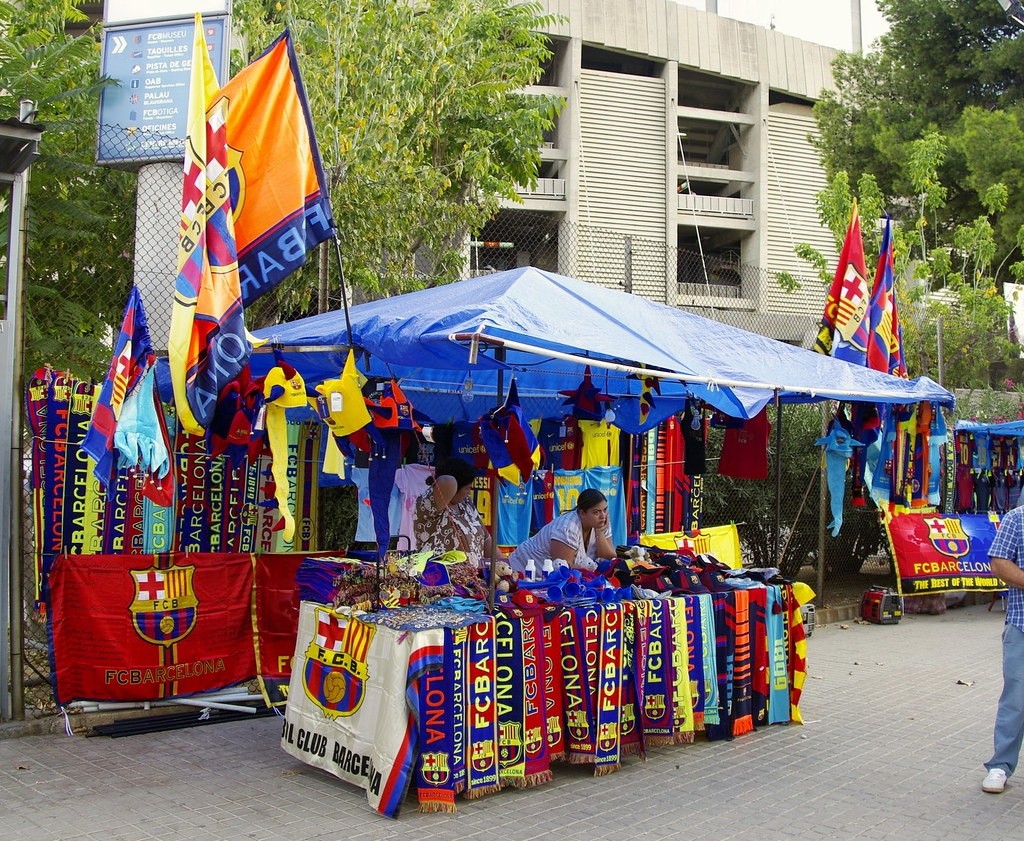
[982,767,1006,792]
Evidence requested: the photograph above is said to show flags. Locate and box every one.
[80,283,157,501]
[209,37,336,305]
[865,216,909,381]
[813,197,871,367]
[167,12,248,437]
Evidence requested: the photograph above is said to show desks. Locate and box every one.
[279,581,817,821]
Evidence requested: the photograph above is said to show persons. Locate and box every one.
[409,453,503,564]
[981,504,1024,796]
[504,488,618,582]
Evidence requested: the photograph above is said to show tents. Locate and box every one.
[957,414,1024,437]
[241,265,956,609]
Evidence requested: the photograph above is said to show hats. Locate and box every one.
[472,374,545,489]
[486,589,515,608]
[307,349,386,468]
[815,420,867,538]
[593,550,736,593]
[208,361,267,466]
[509,589,540,610]
[556,365,616,422]
[363,381,422,559]
[263,360,307,544]
